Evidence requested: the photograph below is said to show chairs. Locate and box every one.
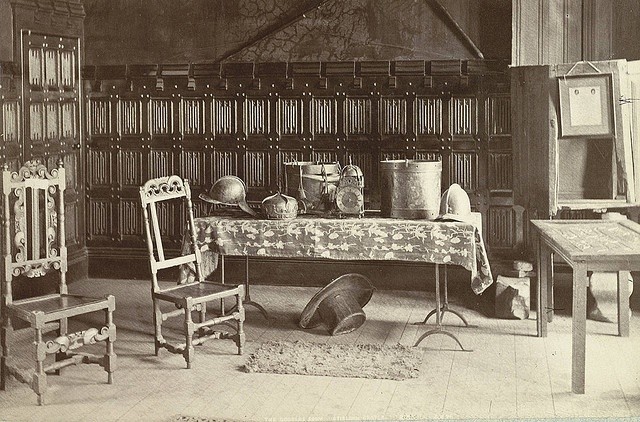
[1,160,117,405]
[139,174,247,368]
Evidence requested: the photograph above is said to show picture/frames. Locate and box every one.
[555,71,617,140]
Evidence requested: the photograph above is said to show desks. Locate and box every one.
[178,214,494,352]
[531,218,640,393]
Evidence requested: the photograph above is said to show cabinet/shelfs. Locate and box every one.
[506,57,639,274]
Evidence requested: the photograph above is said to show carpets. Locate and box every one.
[239,338,424,381]
[166,413,248,422]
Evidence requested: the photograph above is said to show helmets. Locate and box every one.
[261,193,298,219]
[434,184,471,222]
[199,175,260,220]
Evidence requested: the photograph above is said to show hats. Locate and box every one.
[300,273,377,336]
